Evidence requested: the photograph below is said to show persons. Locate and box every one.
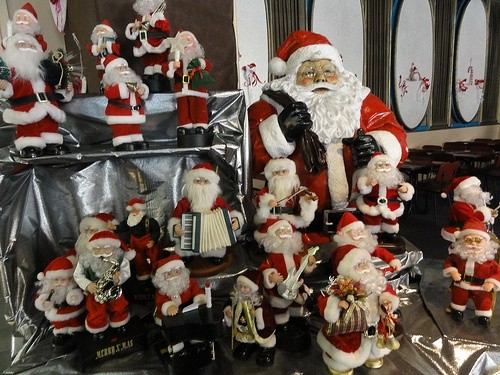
[246,31,408,248]
[0,0,214,157]
[36,151,500,375]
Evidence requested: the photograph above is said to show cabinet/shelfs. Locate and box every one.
[0,90,500,375]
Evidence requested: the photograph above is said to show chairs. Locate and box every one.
[399,138,500,227]
